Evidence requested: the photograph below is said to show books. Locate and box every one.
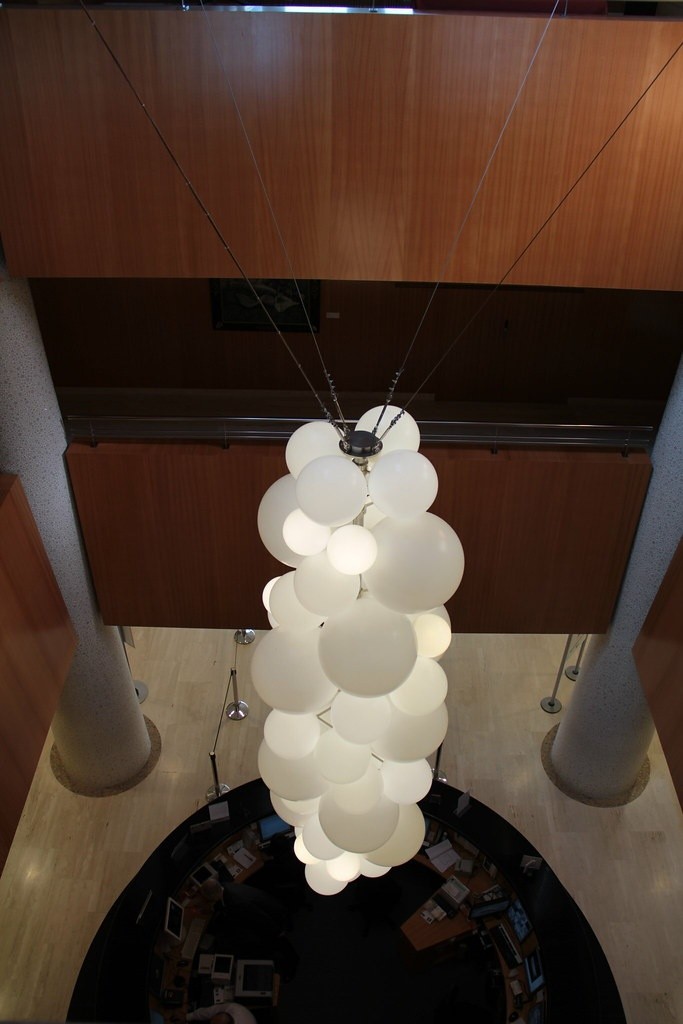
[424,839,459,873]
[225,862,243,879]
[233,848,256,869]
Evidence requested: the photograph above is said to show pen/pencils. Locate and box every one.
[245,855,253,861]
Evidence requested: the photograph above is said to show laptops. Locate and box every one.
[482,860,520,897]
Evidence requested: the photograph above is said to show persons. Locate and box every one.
[172,1003,257,1024]
[202,880,298,984]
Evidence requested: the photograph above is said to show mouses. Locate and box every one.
[177,960,188,967]
[508,1012,519,1022]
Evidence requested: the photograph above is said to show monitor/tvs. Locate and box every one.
[163,897,186,944]
[467,896,512,924]
[249,811,291,843]
[505,897,535,943]
[524,947,547,995]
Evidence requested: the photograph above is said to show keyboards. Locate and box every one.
[259,834,290,850]
[490,923,522,969]
[181,918,205,960]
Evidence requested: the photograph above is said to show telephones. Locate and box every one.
[158,989,183,1008]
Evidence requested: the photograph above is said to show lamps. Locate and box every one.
[76,1,683,899]
